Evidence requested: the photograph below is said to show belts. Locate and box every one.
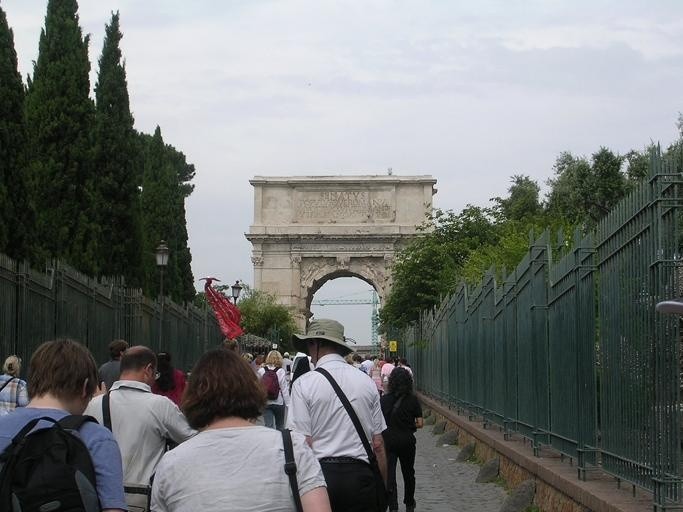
[124,485,150,495]
[322,456,359,464]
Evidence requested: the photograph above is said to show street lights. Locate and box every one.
[231,280,243,305]
[155,240,169,352]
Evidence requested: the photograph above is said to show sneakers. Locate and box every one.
[405,499,416,512]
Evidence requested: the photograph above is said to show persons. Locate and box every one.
[1,319,423,512]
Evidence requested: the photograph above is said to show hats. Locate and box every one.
[292,319,354,358]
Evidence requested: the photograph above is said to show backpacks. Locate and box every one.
[261,367,282,400]
[382,393,409,450]
[0,415,104,511]
